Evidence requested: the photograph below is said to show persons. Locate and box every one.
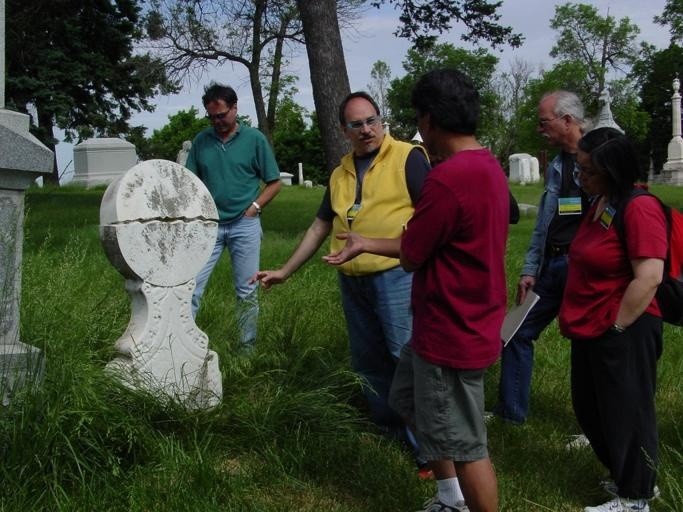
[250,92,428,433]
[323,70,509,512]
[497,90,588,426]
[184,85,281,359]
[564,127,666,512]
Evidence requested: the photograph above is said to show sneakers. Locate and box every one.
[599,478,660,501]
[420,495,471,512]
[566,433,589,452]
[584,495,650,512]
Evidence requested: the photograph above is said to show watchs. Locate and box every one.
[252,201,263,214]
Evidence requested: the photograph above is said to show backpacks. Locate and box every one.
[619,191,683,334]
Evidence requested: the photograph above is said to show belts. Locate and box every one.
[546,241,570,256]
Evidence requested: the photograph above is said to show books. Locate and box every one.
[500,289,540,346]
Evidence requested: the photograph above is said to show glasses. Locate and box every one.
[537,119,550,128]
[347,115,379,130]
[573,161,593,177]
[204,108,232,120]
